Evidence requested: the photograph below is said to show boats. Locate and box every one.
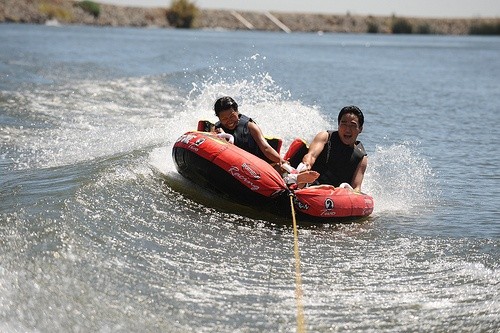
[172,120,374,220]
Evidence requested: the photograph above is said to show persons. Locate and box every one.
[286,105,368,193]
[209,96,291,174]
